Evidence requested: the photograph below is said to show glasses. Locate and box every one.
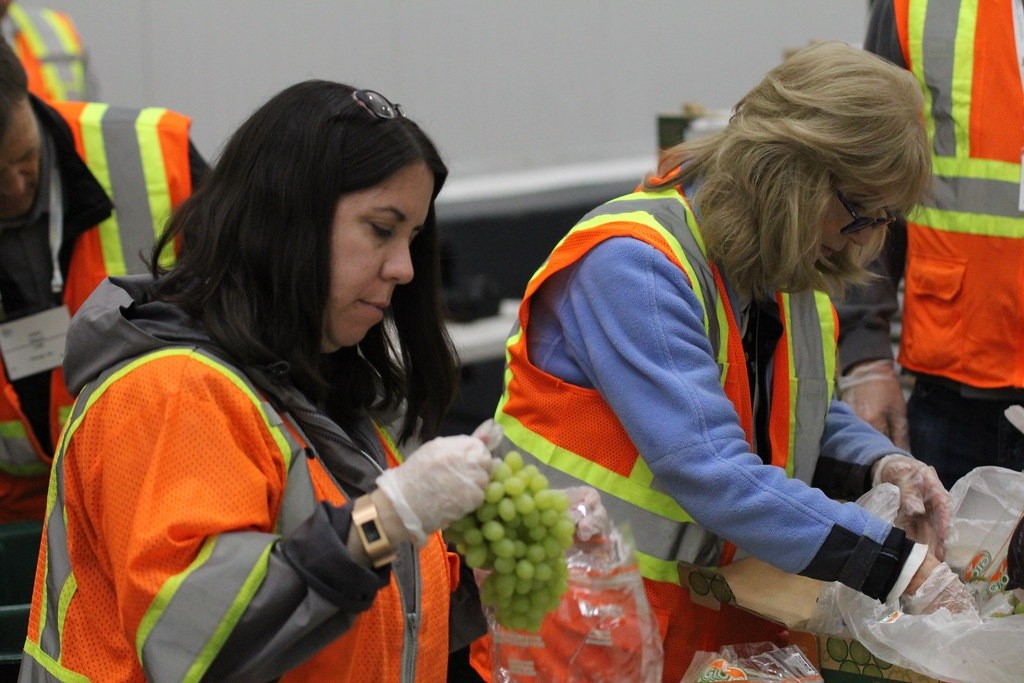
[831,186,896,235]
[321,89,406,121]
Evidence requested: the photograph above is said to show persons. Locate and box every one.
[1,0,103,107]
[445,40,972,682]
[16,78,611,683]
[0,36,212,529]
[828,0,1024,593]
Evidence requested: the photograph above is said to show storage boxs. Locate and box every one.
[678,553,947,683]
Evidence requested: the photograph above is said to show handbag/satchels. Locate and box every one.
[944,466,1024,594]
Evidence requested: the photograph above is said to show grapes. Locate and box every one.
[991,594,1024,618]
[442,451,575,635]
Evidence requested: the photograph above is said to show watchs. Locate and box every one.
[350,491,398,569]
